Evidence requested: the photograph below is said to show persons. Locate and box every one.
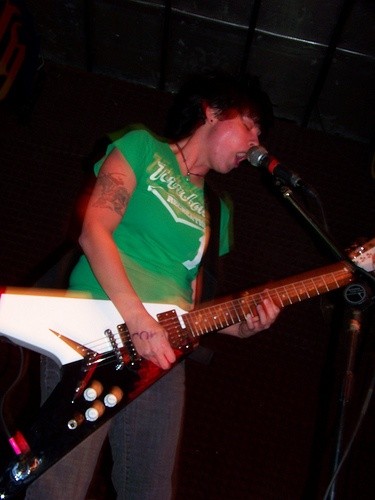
[25,62,274,500]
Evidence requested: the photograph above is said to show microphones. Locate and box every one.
[247,145,318,197]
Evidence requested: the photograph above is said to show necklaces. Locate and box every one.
[175,141,206,182]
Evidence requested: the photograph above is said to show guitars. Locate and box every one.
[0,238,374,496]
